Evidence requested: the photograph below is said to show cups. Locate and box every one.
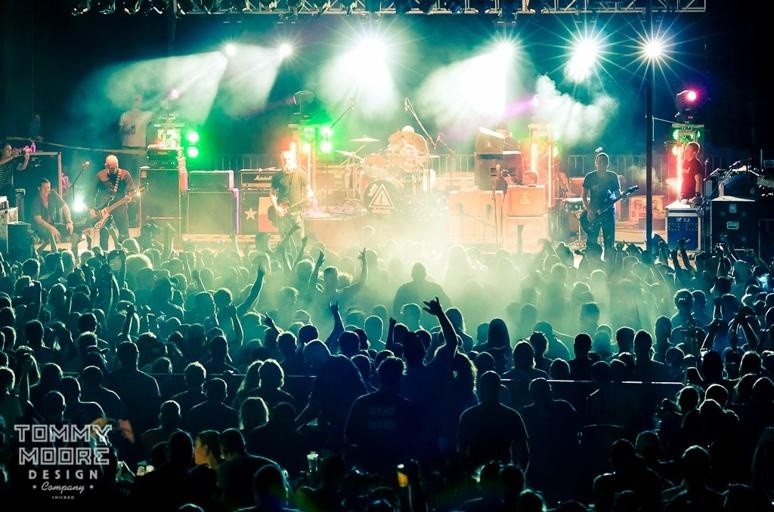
[306,455,319,472]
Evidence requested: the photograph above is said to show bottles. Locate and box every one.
[396,463,409,488]
[718,181,725,198]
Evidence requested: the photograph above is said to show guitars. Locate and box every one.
[579,185,640,234]
[90,184,149,229]
[268,188,327,223]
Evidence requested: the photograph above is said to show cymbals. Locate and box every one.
[417,155,439,159]
[351,137,379,142]
[338,150,364,162]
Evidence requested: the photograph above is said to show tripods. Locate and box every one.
[307,107,367,216]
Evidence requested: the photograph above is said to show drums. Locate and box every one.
[361,176,402,218]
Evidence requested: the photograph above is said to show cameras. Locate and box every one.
[14,149,23,155]
[156,314,166,322]
[29,282,36,288]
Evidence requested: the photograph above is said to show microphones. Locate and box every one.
[496,164,501,180]
[404,97,407,111]
[351,99,354,110]
[82,161,90,170]
[729,160,741,168]
[282,163,288,173]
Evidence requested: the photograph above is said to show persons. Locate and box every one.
[1,125,774,512]
[116,93,155,149]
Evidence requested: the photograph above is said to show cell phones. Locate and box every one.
[700,348,709,362]
